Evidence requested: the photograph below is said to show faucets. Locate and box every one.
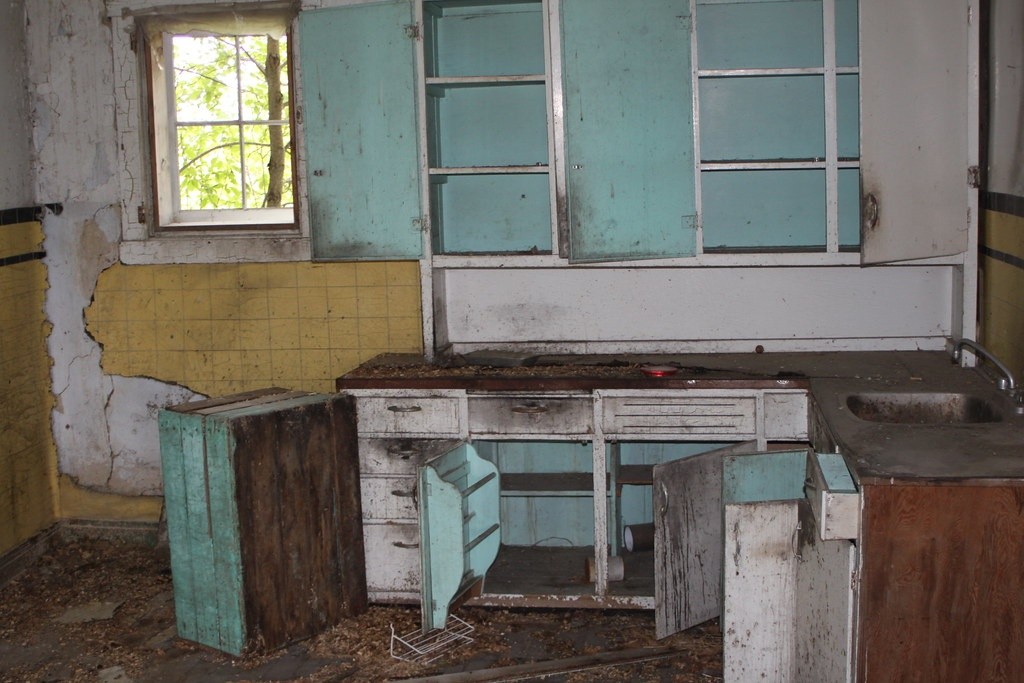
[950,339,1015,391]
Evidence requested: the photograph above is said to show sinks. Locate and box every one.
[845,390,1005,426]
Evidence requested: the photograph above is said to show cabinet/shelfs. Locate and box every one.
[156,384,369,660]
[335,352,808,642]
[413,0,980,366]
[794,446,859,683]
